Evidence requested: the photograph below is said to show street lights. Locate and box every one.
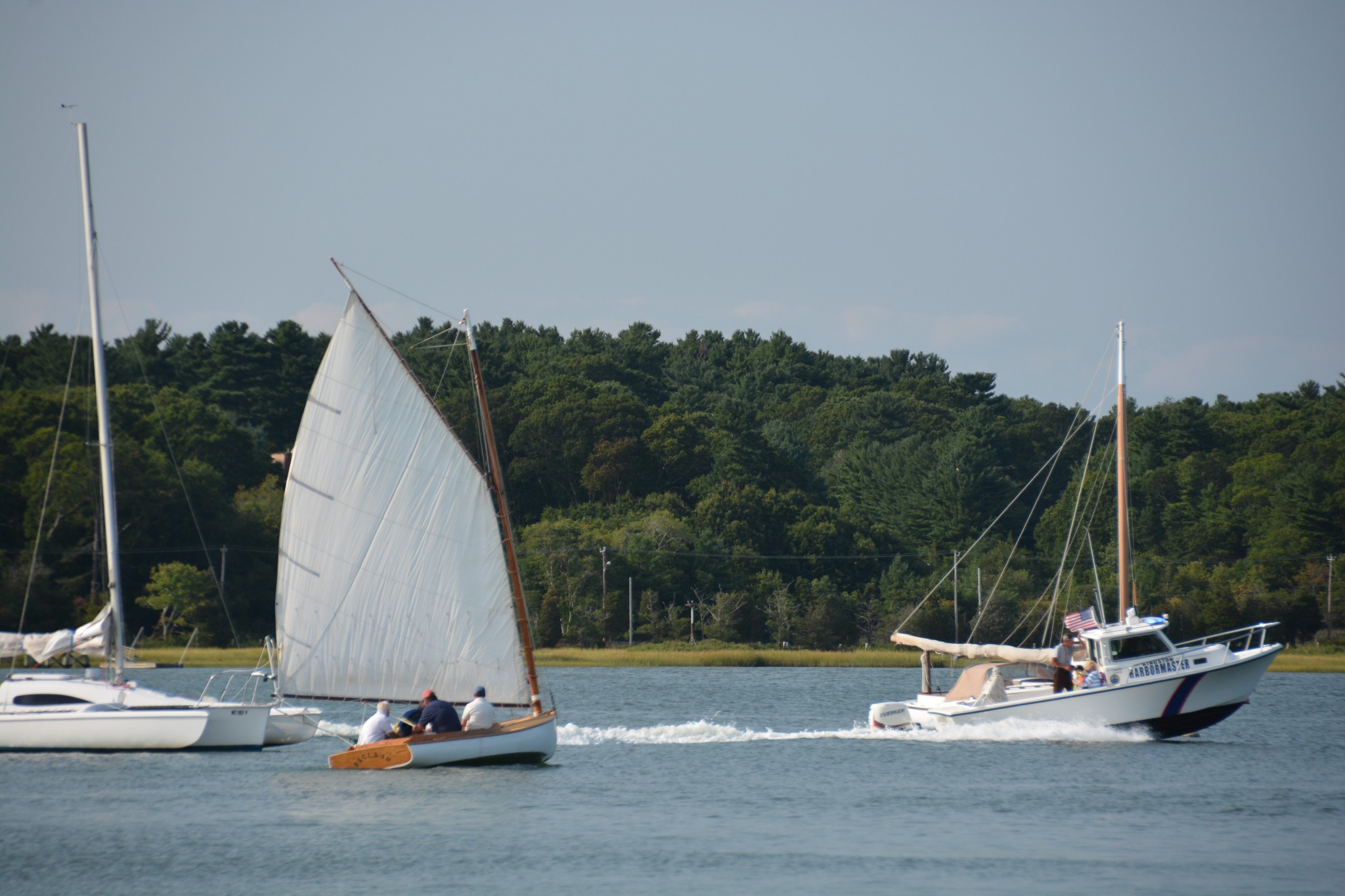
[603,561,611,645]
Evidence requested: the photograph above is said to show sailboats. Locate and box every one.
[1,104,324,752]
[275,257,559,772]
[869,322,1284,742]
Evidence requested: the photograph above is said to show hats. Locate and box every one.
[474,686,485,696]
[1074,665,1084,670]
[421,690,434,700]
[376,702,390,712]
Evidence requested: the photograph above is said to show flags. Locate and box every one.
[1064,607,1099,633]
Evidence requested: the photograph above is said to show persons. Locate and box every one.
[412,689,461,739]
[461,686,495,731]
[357,701,401,746]
[1050,633,1104,695]
[397,699,426,738]
